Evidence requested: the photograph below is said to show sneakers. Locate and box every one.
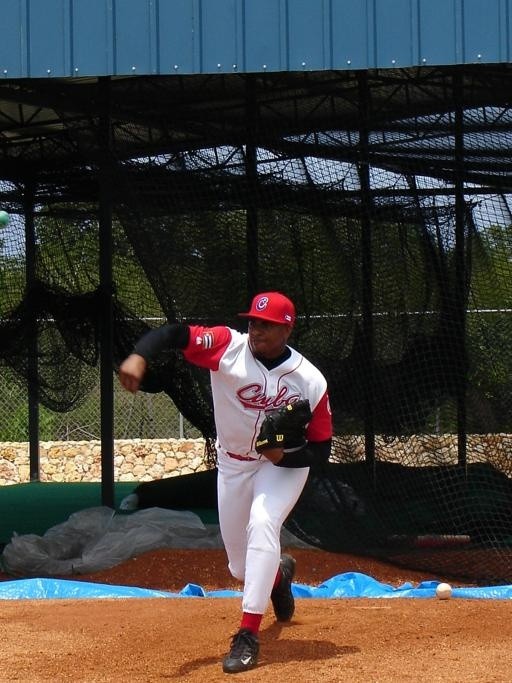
[270,554,296,622]
[224,629,258,673]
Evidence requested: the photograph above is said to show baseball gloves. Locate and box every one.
[255,399,312,454]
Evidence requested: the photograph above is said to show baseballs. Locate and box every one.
[436,583,452,600]
[0,211,10,229]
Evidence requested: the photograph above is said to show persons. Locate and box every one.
[120,290,336,675]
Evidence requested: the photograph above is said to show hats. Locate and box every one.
[237,292,296,326]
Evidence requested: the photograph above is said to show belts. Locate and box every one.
[219,444,257,461]
